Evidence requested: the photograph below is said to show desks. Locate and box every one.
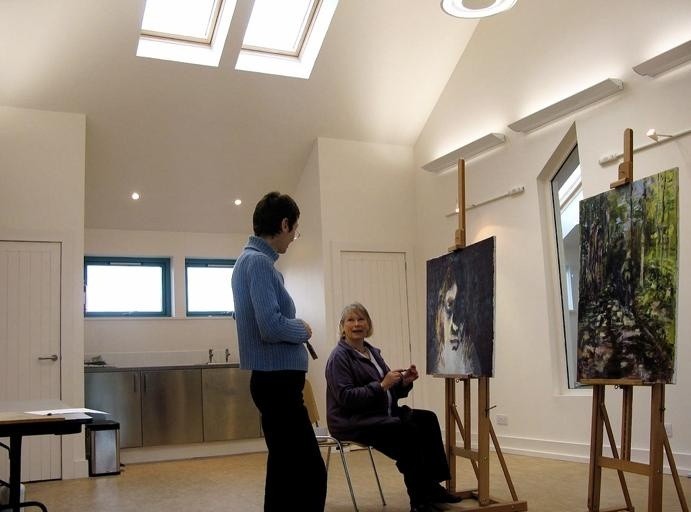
[0,398,95,512]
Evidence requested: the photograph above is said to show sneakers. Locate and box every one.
[437,484,463,504]
[409,504,446,512]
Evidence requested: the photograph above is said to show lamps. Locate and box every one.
[440,0,518,21]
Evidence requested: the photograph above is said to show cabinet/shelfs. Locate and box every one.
[84,365,203,456]
[201,363,266,443]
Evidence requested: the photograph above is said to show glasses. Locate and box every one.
[294,229,302,241]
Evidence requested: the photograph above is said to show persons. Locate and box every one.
[325,302,463,510]
[232,191,327,512]
[432,262,490,373]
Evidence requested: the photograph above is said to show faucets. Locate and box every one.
[208,348,215,363]
[224,348,231,362]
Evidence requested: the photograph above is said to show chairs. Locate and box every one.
[302,379,387,511]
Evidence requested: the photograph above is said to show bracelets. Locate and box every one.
[380,380,387,392]
